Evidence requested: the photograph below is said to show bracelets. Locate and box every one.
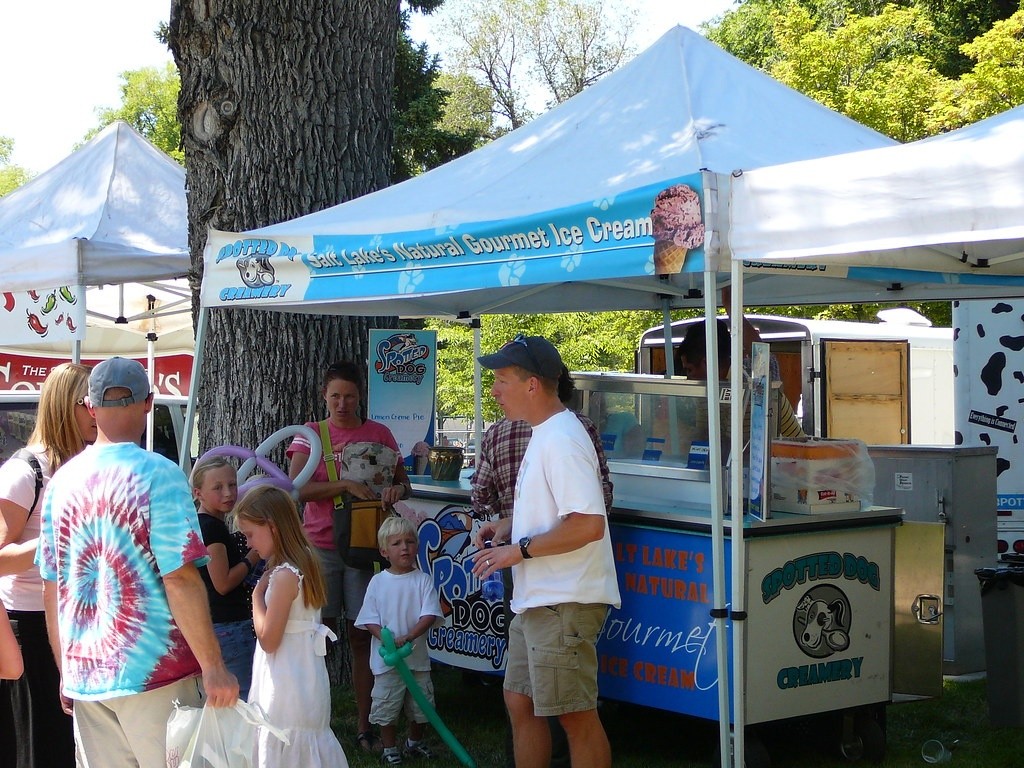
[393,482,407,492]
[241,558,252,572]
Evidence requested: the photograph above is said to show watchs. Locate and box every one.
[519,537,533,559]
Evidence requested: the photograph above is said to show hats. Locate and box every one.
[89,357,150,408]
[477,336,561,379]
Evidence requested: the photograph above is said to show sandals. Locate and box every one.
[357,731,373,752]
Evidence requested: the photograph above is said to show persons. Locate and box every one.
[681,320,804,438]
[0,362,98,768]
[286,361,412,752]
[470,365,614,768]
[353,517,446,764]
[36,356,240,768]
[476,337,621,768]
[192,456,257,703]
[232,484,350,768]
[721,285,779,380]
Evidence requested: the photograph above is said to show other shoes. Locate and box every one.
[403,740,432,760]
[384,746,402,764]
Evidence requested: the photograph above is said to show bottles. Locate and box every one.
[429,447,464,480]
[482,540,505,602]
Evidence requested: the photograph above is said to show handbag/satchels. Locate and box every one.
[166,699,202,768]
[180,698,290,768]
[333,500,400,570]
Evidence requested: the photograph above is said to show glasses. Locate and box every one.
[513,334,545,380]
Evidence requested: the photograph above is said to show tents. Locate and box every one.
[180,23,1024,768]
[0,119,192,452]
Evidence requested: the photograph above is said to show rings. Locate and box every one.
[486,560,490,566]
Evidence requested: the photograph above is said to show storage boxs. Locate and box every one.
[767,435,866,516]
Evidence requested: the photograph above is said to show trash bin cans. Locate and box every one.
[974,554,1024,730]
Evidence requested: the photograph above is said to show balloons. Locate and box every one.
[378,625,476,768]
[200,425,322,501]
